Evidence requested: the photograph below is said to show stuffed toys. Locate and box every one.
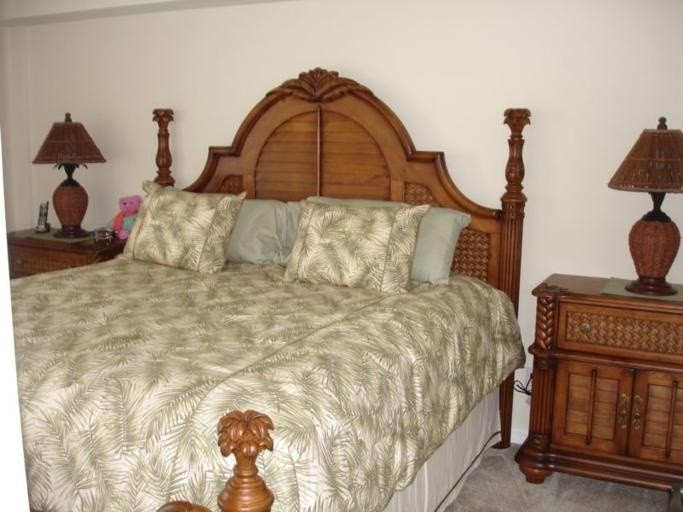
[108,195,143,240]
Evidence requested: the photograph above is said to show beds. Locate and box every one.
[0,67,532,511]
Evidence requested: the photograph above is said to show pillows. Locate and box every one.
[285,198,433,293]
[123,180,248,274]
[226,199,285,266]
[274,197,472,287]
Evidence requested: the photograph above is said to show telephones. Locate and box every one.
[35,201,50,232]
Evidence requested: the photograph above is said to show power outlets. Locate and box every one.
[524,367,531,389]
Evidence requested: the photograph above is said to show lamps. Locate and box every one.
[608,116,683,295]
[32,113,107,238]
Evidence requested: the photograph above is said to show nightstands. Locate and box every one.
[514,274,683,512]
[7,228,127,279]
